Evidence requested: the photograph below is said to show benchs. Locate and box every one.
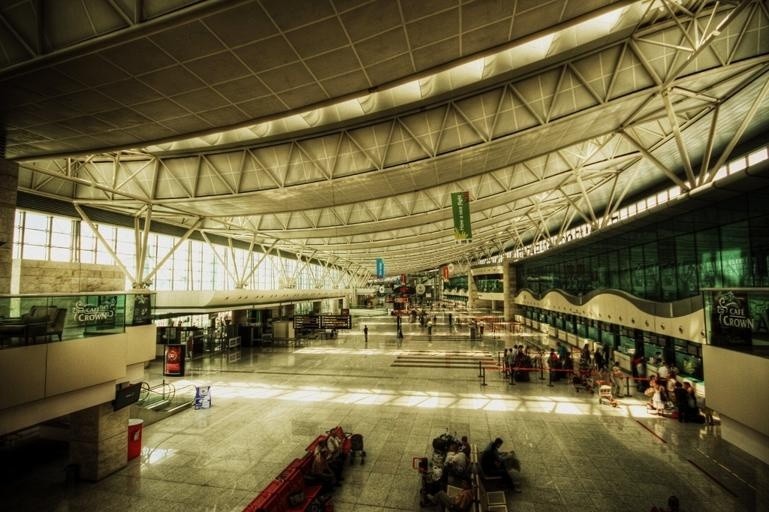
[242,426,355,512]
[444,443,509,512]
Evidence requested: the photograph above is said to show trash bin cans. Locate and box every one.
[128,419,144,460]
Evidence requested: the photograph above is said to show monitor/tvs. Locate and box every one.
[113,382,142,412]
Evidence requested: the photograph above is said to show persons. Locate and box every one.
[410,310,432,336]
[363,325,368,342]
[667,494,682,511]
[418,435,523,511]
[500,339,700,424]
[310,428,348,493]
[448,313,452,325]
[478,319,484,334]
[186,336,192,357]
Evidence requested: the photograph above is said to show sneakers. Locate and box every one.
[513,481,520,486]
[427,494,434,501]
[514,486,520,493]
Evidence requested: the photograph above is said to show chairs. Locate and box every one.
[0,304,68,347]
